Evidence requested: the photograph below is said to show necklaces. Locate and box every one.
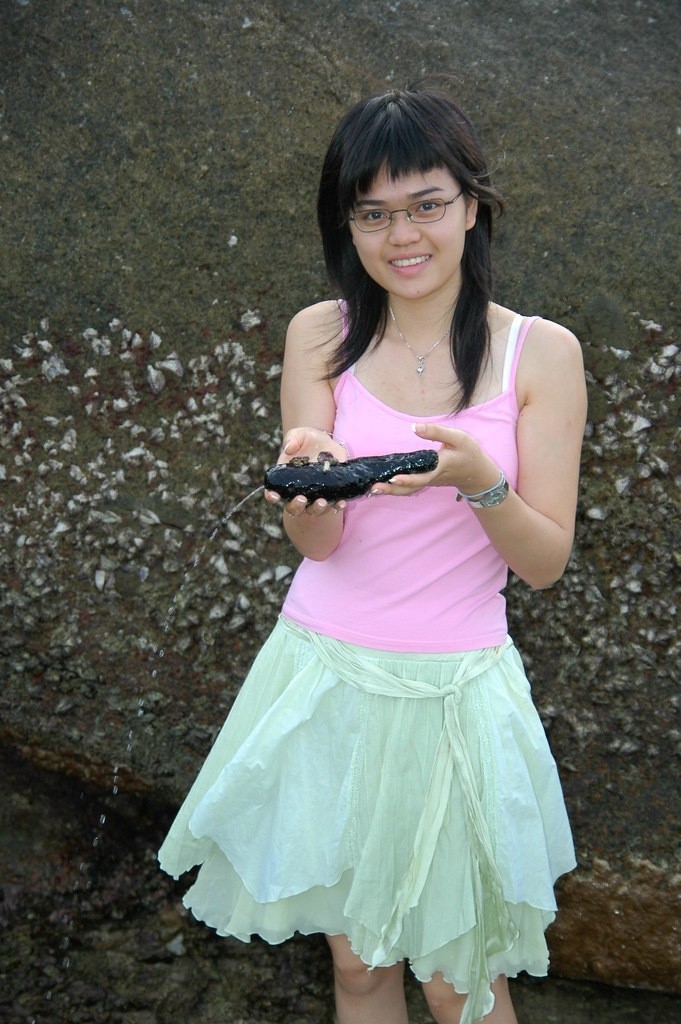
[387,300,450,377]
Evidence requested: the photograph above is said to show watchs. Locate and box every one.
[455,476,510,508]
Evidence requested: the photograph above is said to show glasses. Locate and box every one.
[346,190,465,232]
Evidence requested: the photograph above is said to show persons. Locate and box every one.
[261,89,587,1024]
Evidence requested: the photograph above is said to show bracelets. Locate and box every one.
[322,431,351,460]
[457,469,504,498]
